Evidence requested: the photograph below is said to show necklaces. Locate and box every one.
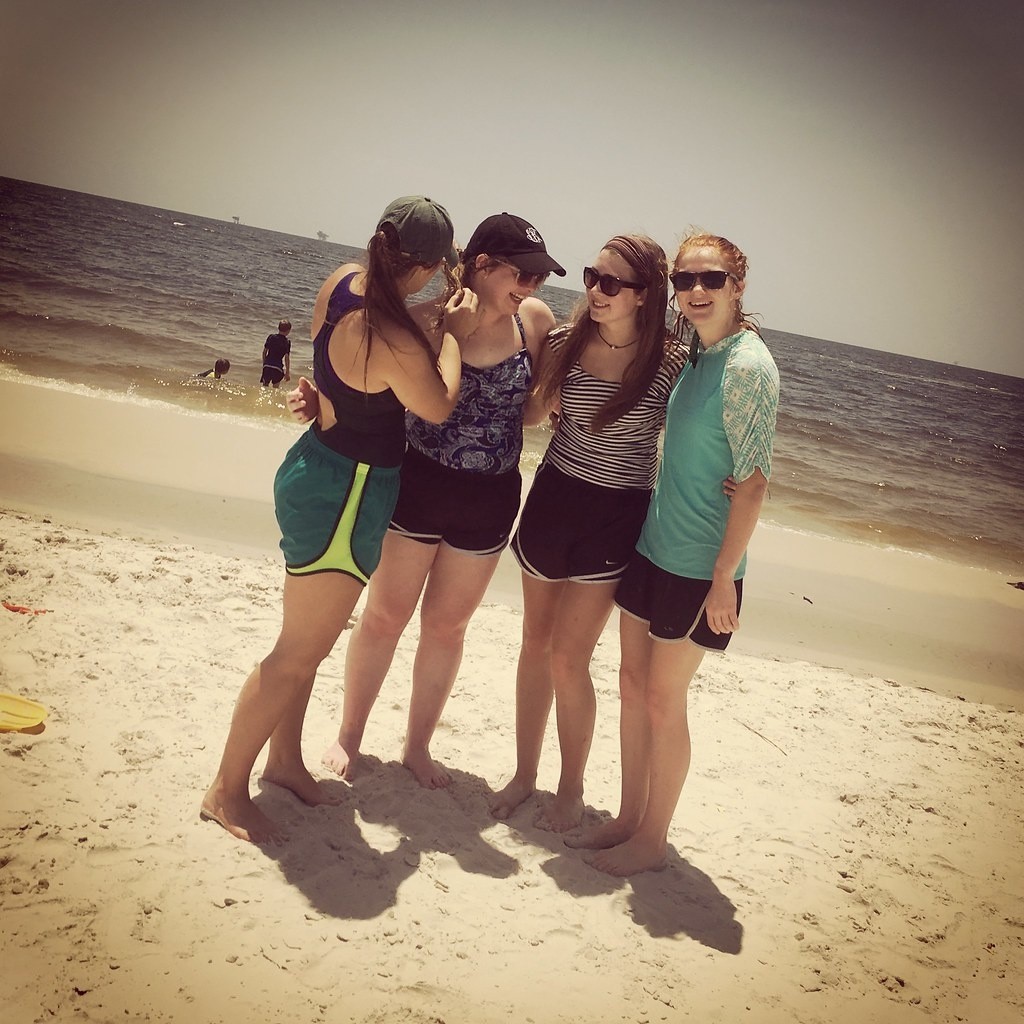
[598,322,640,349]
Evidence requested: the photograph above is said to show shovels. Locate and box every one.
[0,692,47,730]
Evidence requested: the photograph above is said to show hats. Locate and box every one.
[465,211,567,277]
[374,194,460,268]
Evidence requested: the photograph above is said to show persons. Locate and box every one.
[486,235,738,832]
[198,358,230,380]
[202,198,485,849]
[259,320,291,387]
[288,211,559,789]
[566,236,778,874]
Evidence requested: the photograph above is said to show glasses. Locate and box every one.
[583,266,646,297]
[669,270,738,291]
[491,256,551,290]
